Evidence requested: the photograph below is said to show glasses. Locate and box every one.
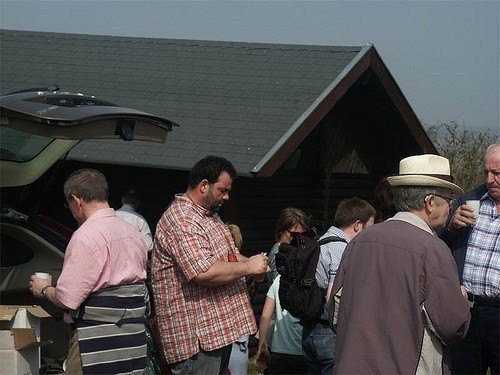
[424,192,458,210]
[64,198,70,209]
[286,228,305,238]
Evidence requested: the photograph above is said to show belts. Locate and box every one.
[467,292,500,308]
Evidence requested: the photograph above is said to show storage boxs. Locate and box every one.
[0,304,53,375]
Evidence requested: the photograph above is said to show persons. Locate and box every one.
[434,143,500,374]
[252,175,404,375]
[28,167,155,375]
[149,154,272,375]
[325,153,474,375]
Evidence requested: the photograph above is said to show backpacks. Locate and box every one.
[274,229,349,329]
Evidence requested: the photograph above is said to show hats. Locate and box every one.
[386,153,465,196]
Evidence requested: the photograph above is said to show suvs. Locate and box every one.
[0,83,181,303]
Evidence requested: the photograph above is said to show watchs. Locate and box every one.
[42,285,53,297]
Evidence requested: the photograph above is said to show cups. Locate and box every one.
[466,200,479,223]
[35,273,49,280]
